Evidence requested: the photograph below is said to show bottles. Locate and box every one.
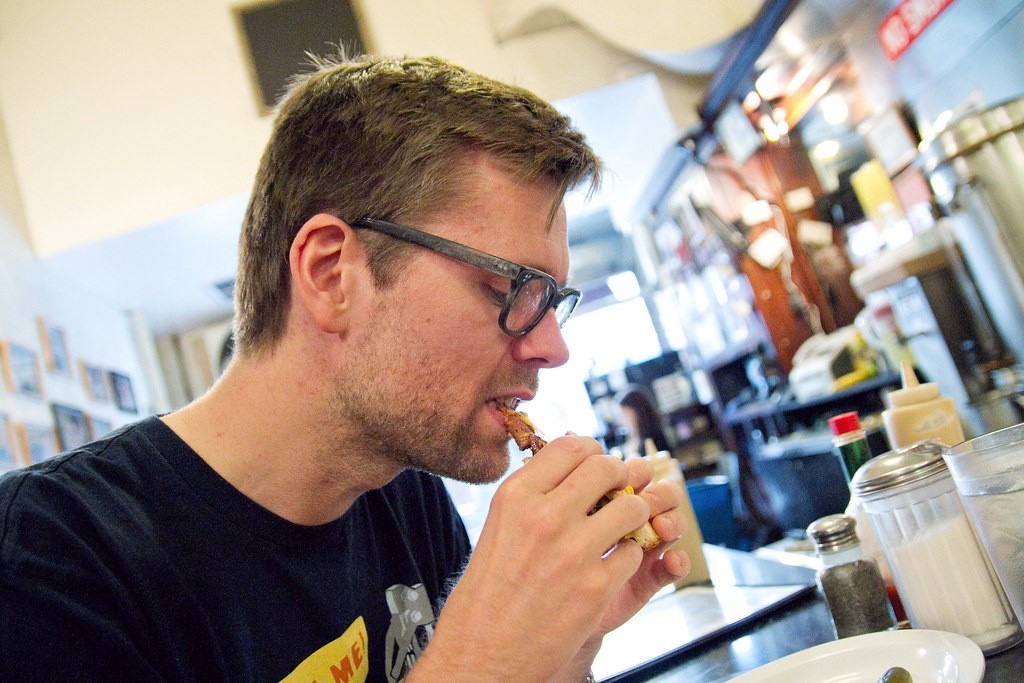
[640,437,710,588]
[805,512,900,640]
[882,358,964,451]
[826,411,912,630]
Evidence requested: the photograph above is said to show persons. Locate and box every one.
[0,44,692,683]
[618,391,673,456]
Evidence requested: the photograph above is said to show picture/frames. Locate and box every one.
[79,362,108,402]
[36,317,73,378]
[0,343,44,397]
[51,403,89,452]
[110,372,137,413]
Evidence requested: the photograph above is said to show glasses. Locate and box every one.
[339,214,582,337]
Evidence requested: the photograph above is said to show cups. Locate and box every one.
[938,423,1024,634]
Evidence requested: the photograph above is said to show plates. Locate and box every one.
[723,628,986,683]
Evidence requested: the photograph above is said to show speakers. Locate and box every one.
[235,0,369,114]
